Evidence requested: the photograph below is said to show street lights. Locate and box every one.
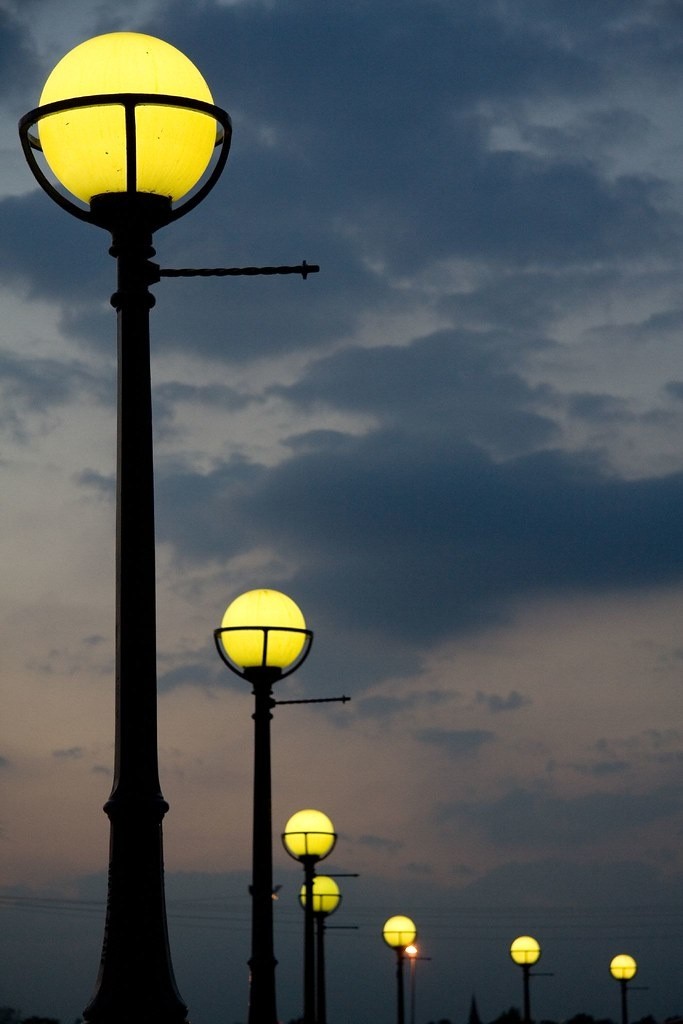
[405,943,418,1024]
[279,809,361,1024]
[14,32,321,1024]
[381,914,433,1024]
[297,875,359,1024]
[212,587,353,1024]
[510,936,554,1024]
[609,954,650,1024]
